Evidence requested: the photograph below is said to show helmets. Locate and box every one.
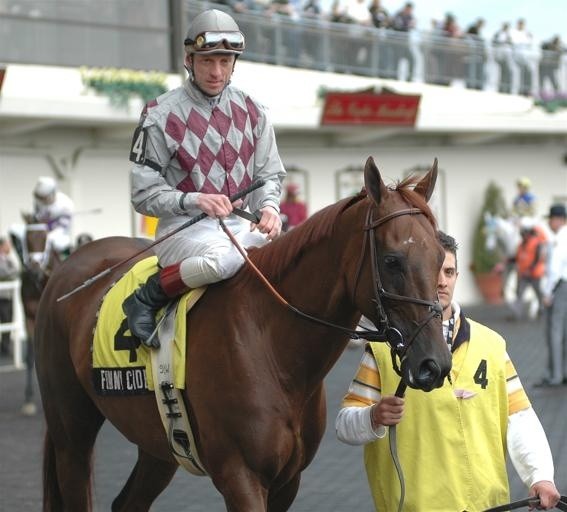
[34,175,54,196]
[183,9,244,57]
[77,233,91,244]
[517,176,528,186]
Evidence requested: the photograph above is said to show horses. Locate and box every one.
[32,155,455,512]
[19,209,61,417]
[482,209,523,319]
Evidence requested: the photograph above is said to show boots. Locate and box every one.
[121,272,169,350]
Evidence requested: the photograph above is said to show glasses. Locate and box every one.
[200,30,245,50]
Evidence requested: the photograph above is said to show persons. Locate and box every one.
[209,0,299,66]
[391,1,417,82]
[336,231,561,512]
[329,0,349,73]
[467,17,486,89]
[122,8,286,349]
[78,234,92,248]
[431,12,459,86]
[279,184,308,233]
[506,224,552,318]
[491,22,513,94]
[538,35,567,98]
[531,204,567,388]
[512,18,534,97]
[348,1,371,75]
[513,177,539,217]
[32,176,74,270]
[1,236,21,282]
[302,0,320,67]
[369,0,390,77]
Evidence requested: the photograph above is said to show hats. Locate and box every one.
[543,205,565,217]
[289,184,297,193]
